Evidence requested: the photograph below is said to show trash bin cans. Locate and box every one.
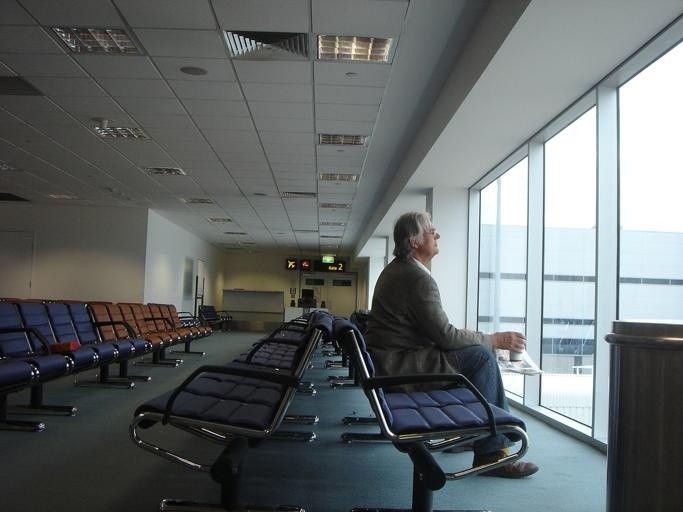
[604,319,683,512]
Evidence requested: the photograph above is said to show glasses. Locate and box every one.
[426,229,437,235]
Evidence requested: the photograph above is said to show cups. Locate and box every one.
[510,350,523,362]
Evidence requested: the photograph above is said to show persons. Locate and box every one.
[361,209,538,479]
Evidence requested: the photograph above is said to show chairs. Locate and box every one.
[0,297,233,433]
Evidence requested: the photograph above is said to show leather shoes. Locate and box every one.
[473,449,539,478]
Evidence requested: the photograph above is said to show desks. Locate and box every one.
[309,307,329,314]
[284,306,304,323]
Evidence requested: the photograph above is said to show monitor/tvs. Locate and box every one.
[302,288,313,300]
